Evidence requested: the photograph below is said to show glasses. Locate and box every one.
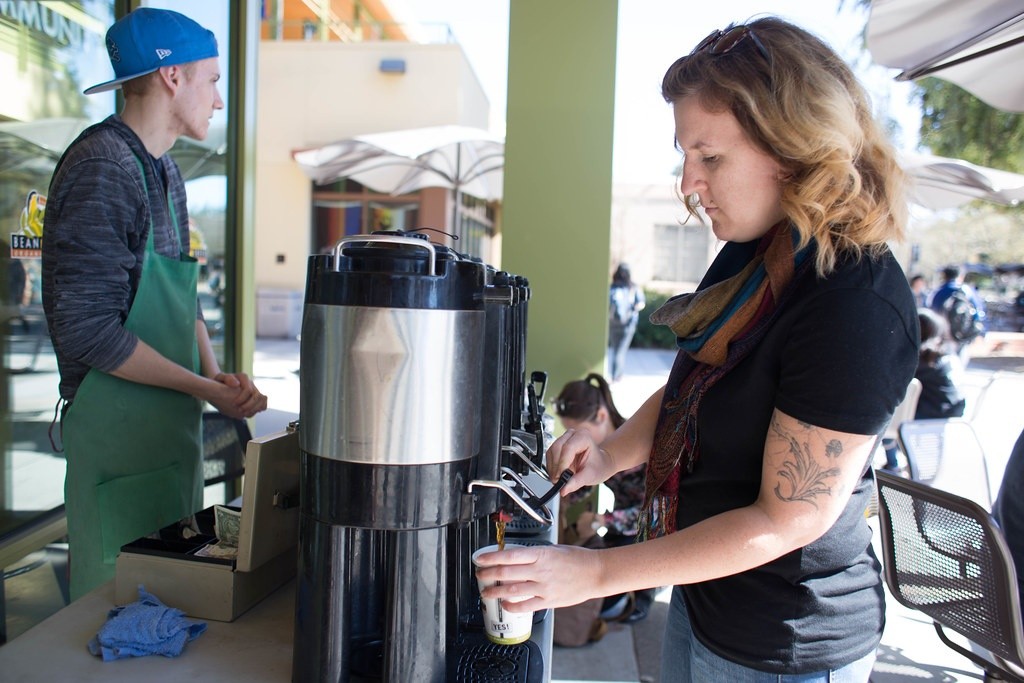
[689,25,773,64]
[549,398,575,416]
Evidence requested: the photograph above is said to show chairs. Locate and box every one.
[898,416,993,580]
[876,468,1024,683]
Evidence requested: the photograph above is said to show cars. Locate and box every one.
[962,263,1024,337]
[197,280,221,335]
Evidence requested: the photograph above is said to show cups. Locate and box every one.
[471,543,534,644]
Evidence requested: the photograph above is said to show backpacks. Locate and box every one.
[945,286,985,341]
[608,283,634,328]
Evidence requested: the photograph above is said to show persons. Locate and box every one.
[607,261,645,379]
[881,311,969,475]
[557,373,672,624]
[41,8,268,603]
[473,20,916,683]
[910,262,986,339]
[0,239,31,323]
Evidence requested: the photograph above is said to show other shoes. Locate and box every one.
[623,608,646,623]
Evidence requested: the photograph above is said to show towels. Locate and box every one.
[86,582,210,664]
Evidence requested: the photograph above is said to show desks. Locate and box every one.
[0,458,560,683]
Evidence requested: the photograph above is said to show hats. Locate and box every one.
[82,8,219,94]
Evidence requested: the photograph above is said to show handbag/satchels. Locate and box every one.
[552,522,635,647]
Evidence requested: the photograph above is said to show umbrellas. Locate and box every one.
[1,119,226,183]
[290,125,505,256]
